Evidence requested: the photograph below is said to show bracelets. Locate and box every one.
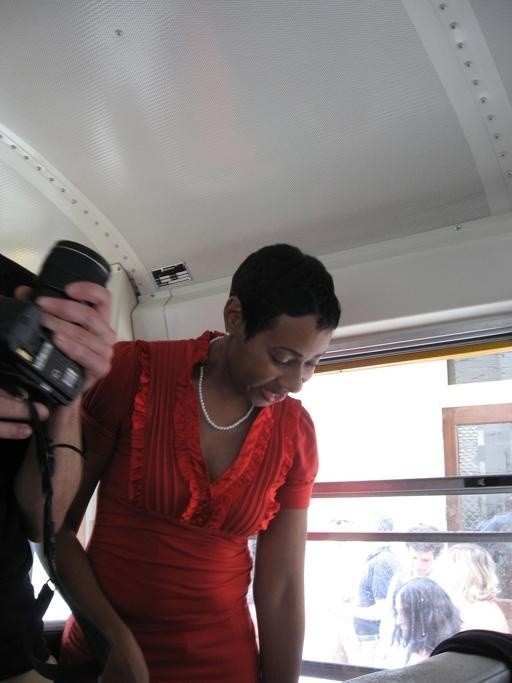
[51,443,85,457]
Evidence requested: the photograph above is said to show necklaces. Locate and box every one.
[199,335,255,429]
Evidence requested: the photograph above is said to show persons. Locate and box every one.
[377,522,447,656]
[387,575,461,666]
[0,244,120,683]
[39,243,341,683]
[444,542,509,634]
[341,516,397,645]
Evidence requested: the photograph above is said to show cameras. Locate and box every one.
[0,239,110,410]
